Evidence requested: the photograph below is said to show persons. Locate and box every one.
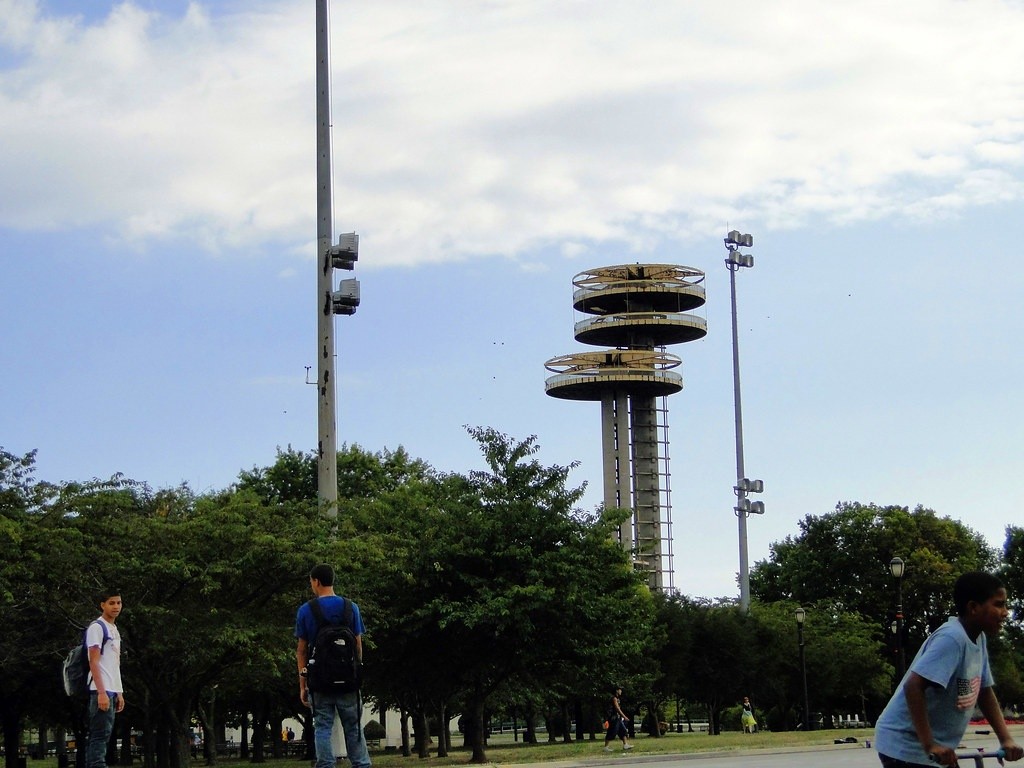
[282,726,295,742]
[603,685,635,752]
[85,587,126,768]
[294,563,374,768]
[873,570,1024,768]
[741,697,757,733]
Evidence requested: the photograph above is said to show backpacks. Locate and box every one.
[300,596,363,696]
[63,619,109,695]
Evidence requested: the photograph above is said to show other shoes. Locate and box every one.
[624,743,634,749]
[603,746,613,752]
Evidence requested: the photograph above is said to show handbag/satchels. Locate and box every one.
[866,738,871,748]
[603,721,609,729]
